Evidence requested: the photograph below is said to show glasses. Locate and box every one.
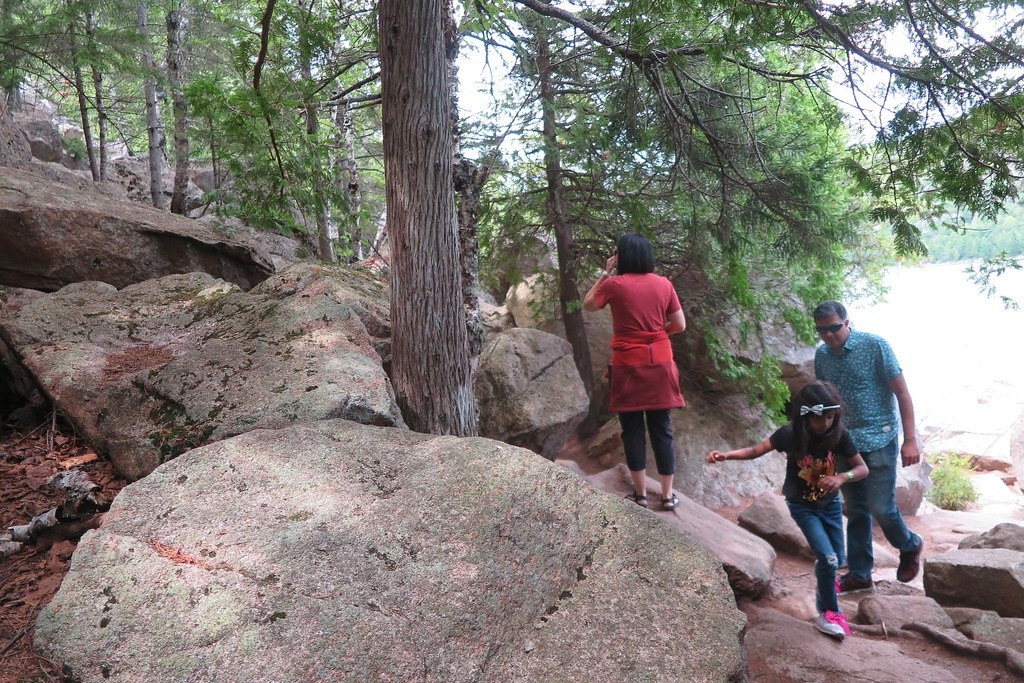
[613,249,618,256]
[816,321,844,334]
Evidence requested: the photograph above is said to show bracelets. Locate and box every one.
[603,271,611,278]
[845,472,855,482]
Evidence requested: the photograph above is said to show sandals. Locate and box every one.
[625,491,648,507]
[662,494,680,509]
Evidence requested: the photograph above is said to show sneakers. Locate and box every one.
[836,573,876,594]
[897,535,924,583]
[814,610,850,639]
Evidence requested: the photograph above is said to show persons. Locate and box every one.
[706,382,868,639]
[584,234,686,510]
[813,301,924,596]
